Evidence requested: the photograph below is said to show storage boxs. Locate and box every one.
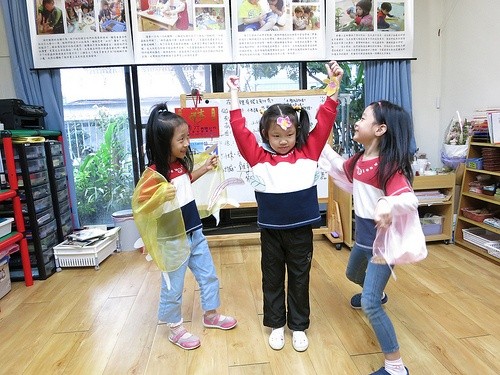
[53,223,120,272]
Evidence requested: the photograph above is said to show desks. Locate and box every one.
[454,107,500,258]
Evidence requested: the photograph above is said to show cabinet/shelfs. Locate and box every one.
[323,162,456,250]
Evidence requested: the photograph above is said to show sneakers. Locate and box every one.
[168,329,200,349]
[292,330,308,352]
[369,364,409,375]
[202,313,236,330]
[349,292,389,309]
[268,326,285,349]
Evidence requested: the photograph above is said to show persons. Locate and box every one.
[226,59,345,353]
[321,99,419,375]
[35,0,403,31]
[135,103,238,350]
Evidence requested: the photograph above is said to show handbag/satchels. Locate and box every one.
[370,195,428,263]
